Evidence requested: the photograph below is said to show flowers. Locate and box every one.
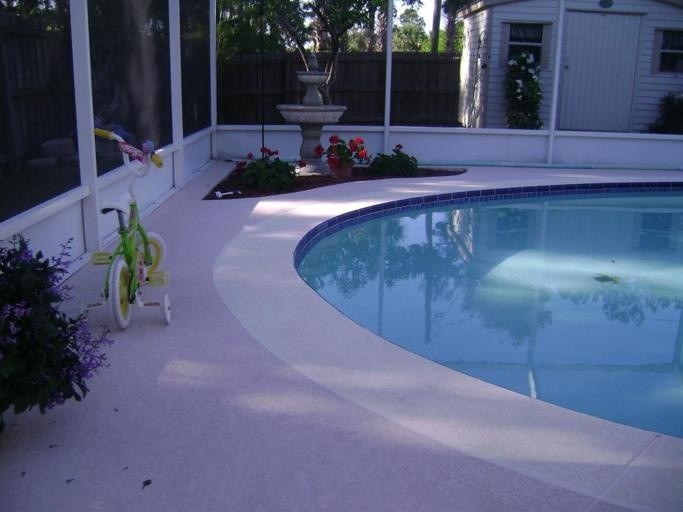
[316,134,372,165]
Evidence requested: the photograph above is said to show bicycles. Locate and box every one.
[85,124,174,332]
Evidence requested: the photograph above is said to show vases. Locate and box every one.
[328,156,355,179]
